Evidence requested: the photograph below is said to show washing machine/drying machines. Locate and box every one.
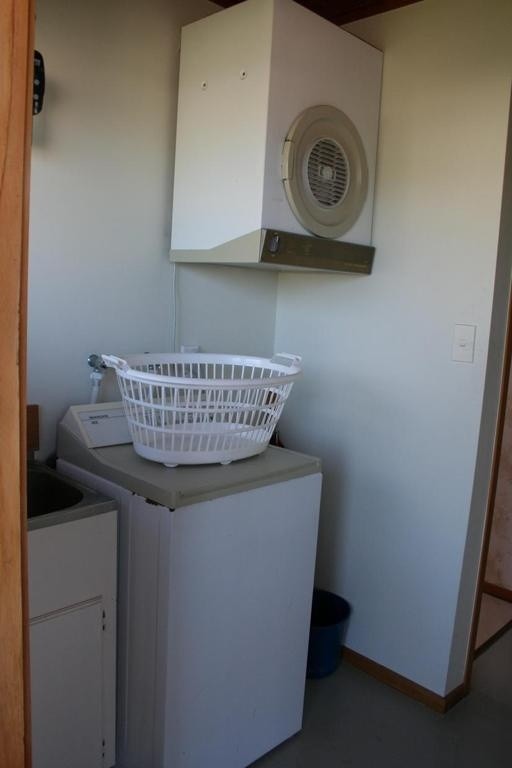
[51,398,324,767]
